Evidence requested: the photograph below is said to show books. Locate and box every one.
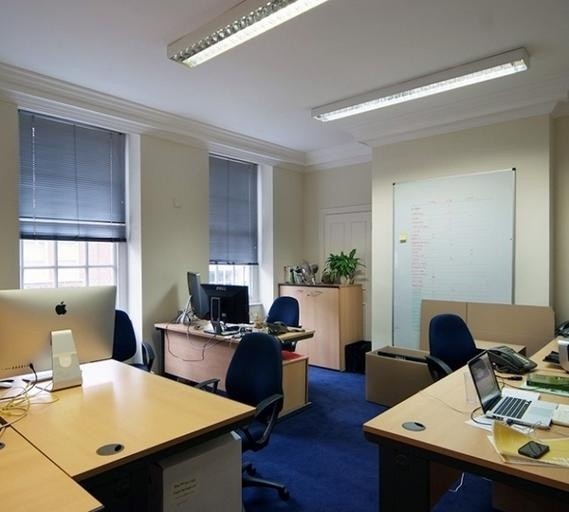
[552,403,569,427]
[526,372,569,391]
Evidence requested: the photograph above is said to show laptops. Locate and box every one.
[467,350,558,429]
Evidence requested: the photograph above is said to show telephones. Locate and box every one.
[486,346,536,374]
[555,320,569,336]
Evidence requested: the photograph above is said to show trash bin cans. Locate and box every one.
[345,341,372,374]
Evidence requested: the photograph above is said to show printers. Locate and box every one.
[543,337,569,373]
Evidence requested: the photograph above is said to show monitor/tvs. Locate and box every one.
[200,283,250,336]
[181,271,201,326]
[0,285,118,392]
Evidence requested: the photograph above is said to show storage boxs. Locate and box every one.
[364,344,431,406]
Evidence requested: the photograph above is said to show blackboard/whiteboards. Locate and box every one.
[392,168,516,347]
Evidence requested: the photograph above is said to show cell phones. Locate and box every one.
[518,441,549,459]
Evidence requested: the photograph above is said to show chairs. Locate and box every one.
[423,313,478,384]
[266,296,300,329]
[114,309,155,373]
[193,335,284,451]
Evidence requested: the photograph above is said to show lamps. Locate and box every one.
[166,1,323,69]
[310,47,530,126]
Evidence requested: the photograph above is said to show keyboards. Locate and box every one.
[22,373,52,383]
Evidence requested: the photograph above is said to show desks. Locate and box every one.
[0,424,105,511]
[155,325,314,419]
[0,361,257,478]
[362,336,569,512]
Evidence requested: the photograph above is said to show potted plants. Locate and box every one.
[325,247,367,287]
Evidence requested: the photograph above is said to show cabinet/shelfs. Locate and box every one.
[278,282,364,373]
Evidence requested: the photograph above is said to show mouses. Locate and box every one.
[0,381,12,388]
